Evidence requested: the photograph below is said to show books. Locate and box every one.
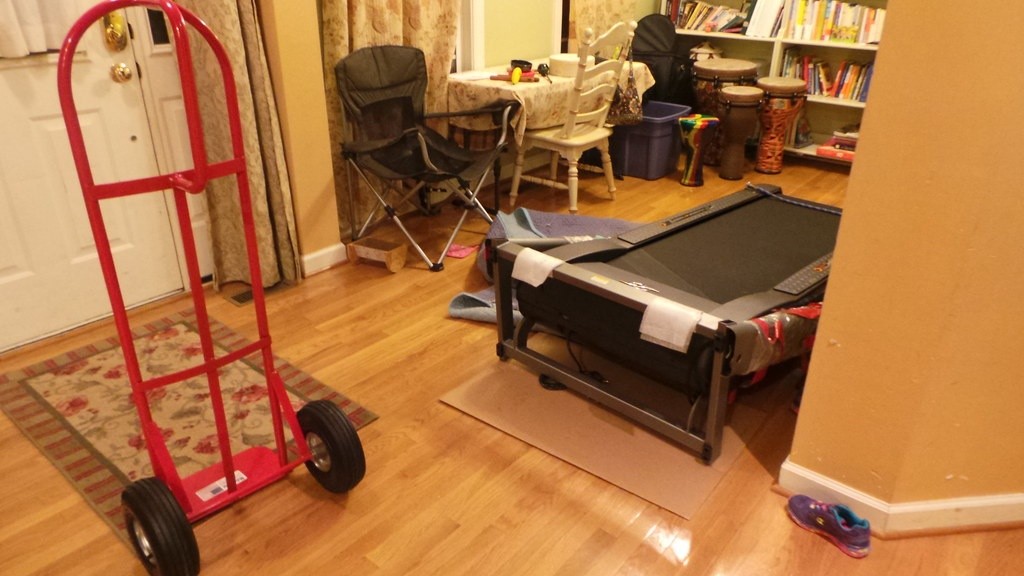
[816,124,859,163]
[779,46,874,105]
[660,0,886,48]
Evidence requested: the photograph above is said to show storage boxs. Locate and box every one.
[579,100,692,180]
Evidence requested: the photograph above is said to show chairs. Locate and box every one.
[510,20,637,213]
[335,45,521,272]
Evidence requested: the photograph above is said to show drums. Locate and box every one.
[679,113,721,187]
[715,83,764,180]
[693,57,757,168]
[756,75,809,175]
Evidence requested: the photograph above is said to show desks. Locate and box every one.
[448,53,655,215]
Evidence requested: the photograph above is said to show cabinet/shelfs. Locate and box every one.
[660,0,888,166]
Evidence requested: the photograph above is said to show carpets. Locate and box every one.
[0,306,380,557]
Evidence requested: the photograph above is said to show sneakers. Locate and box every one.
[788,494,872,558]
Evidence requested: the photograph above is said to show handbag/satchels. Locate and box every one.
[605,44,644,126]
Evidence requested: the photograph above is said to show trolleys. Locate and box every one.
[56,2,367,575]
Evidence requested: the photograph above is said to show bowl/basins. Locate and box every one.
[510,59,532,73]
